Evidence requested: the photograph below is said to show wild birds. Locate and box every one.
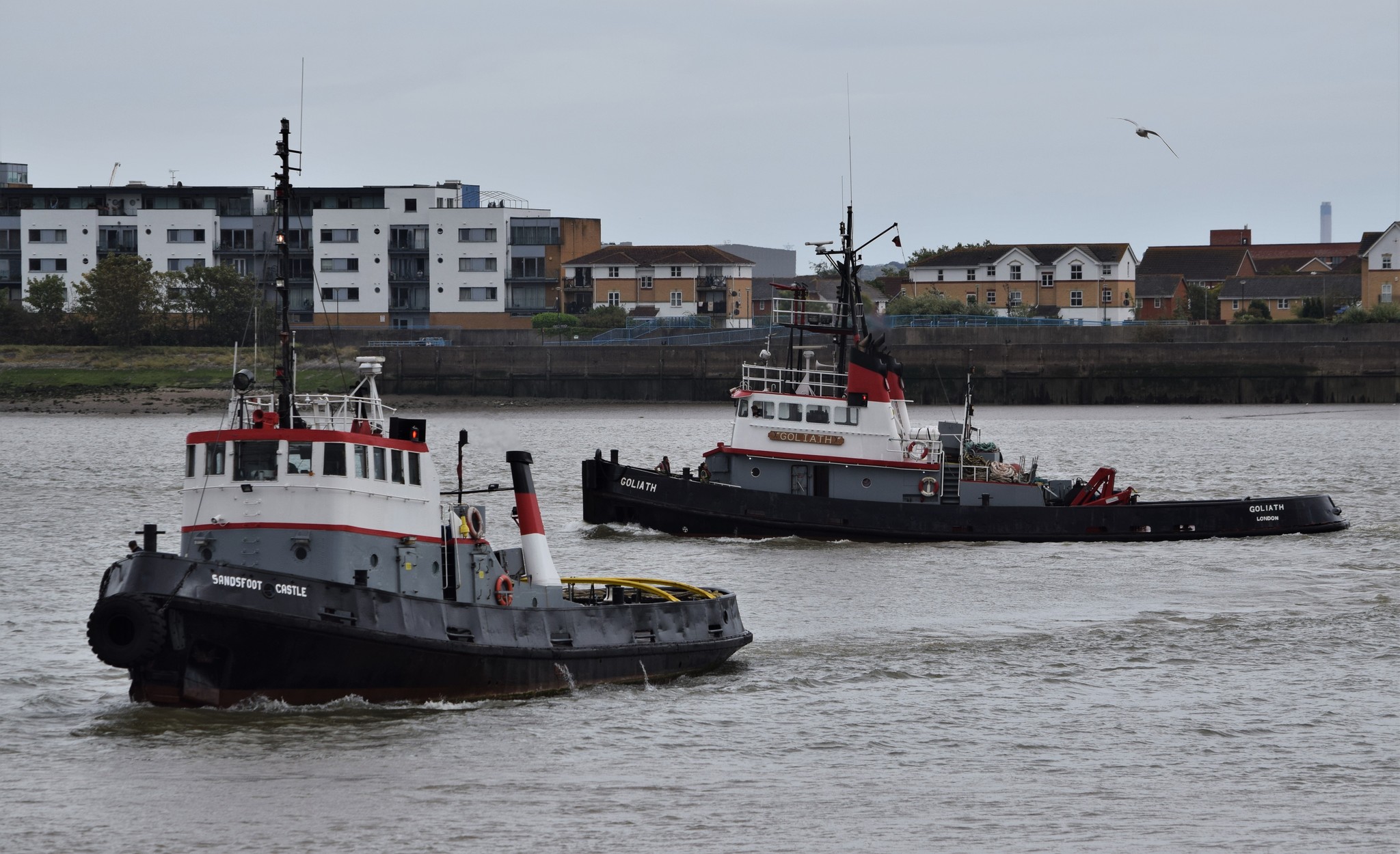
[1105,117,1180,159]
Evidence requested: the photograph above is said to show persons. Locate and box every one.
[700,462,710,484]
[654,456,670,476]
[751,405,768,418]
[128,541,142,552]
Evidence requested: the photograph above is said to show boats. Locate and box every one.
[580,77,1353,543]
[85,54,748,706]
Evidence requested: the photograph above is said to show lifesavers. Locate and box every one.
[467,506,484,539]
[85,593,167,668]
[496,574,513,606]
[908,440,928,460]
[919,477,939,497]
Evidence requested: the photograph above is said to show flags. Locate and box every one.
[892,236,901,247]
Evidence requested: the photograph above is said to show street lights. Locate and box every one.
[710,286,716,327]
[1310,272,1326,322]
[1240,280,1246,317]
[1205,286,1211,321]
[975,284,980,304]
[1100,276,1105,321]
